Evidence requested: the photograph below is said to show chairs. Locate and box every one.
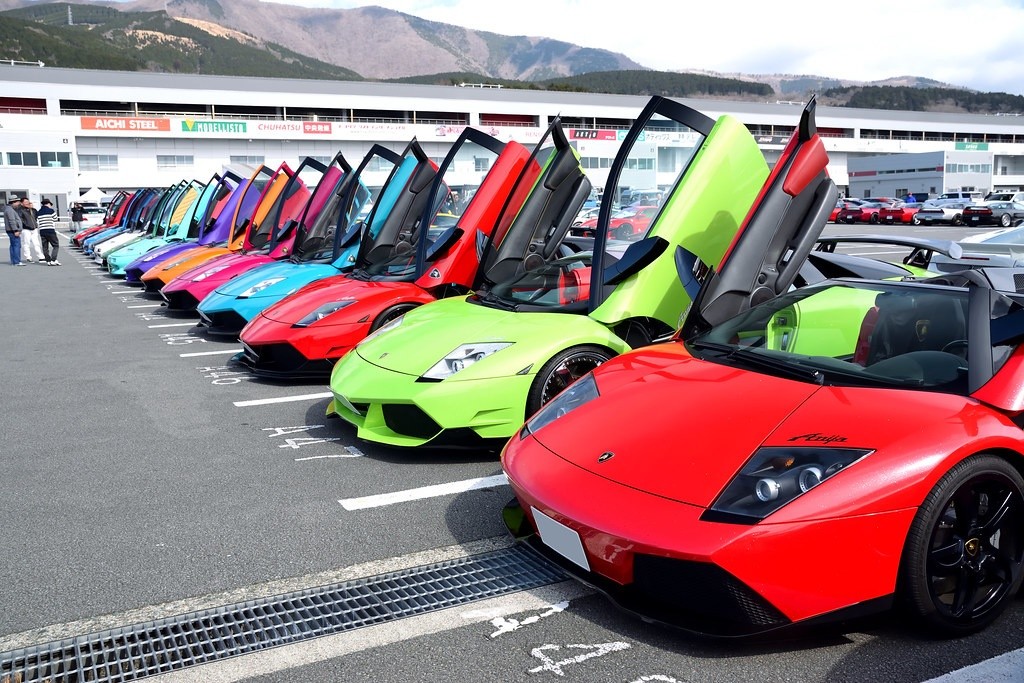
[864,278,967,366]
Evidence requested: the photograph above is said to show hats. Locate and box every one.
[41,199,53,206]
[8,195,20,201]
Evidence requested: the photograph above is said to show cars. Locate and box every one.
[427,213,461,241]
[879,203,925,225]
[965,192,1024,208]
[573,198,662,239]
[924,192,984,207]
[827,197,904,224]
[69,207,112,233]
[961,201,1024,227]
[916,203,970,226]
[839,203,891,224]
[923,225,1024,258]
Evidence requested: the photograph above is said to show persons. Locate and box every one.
[16,198,46,264]
[71,202,88,233]
[28,202,38,222]
[3,194,27,266]
[36,199,62,266]
[905,192,917,203]
[440,192,475,217]
[639,192,668,207]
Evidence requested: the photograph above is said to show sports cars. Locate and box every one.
[229,127,620,379]
[325,95,964,447]
[196,135,435,334]
[68,156,342,312]
[500,93,1024,642]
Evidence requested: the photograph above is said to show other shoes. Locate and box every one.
[39,259,46,263]
[47,262,50,266]
[27,259,35,263]
[12,264,15,266]
[15,262,26,266]
[51,260,61,266]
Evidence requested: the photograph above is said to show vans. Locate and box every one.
[619,189,663,205]
[898,193,929,203]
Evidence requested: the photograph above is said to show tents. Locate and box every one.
[68,185,122,232]
